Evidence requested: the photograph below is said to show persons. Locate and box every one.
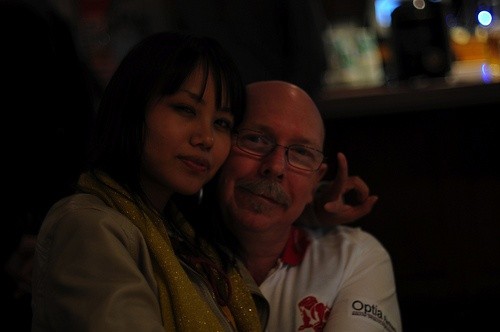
[31,31,379,332]
[18,80,403,332]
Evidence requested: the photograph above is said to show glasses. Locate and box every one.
[233,129,329,172]
